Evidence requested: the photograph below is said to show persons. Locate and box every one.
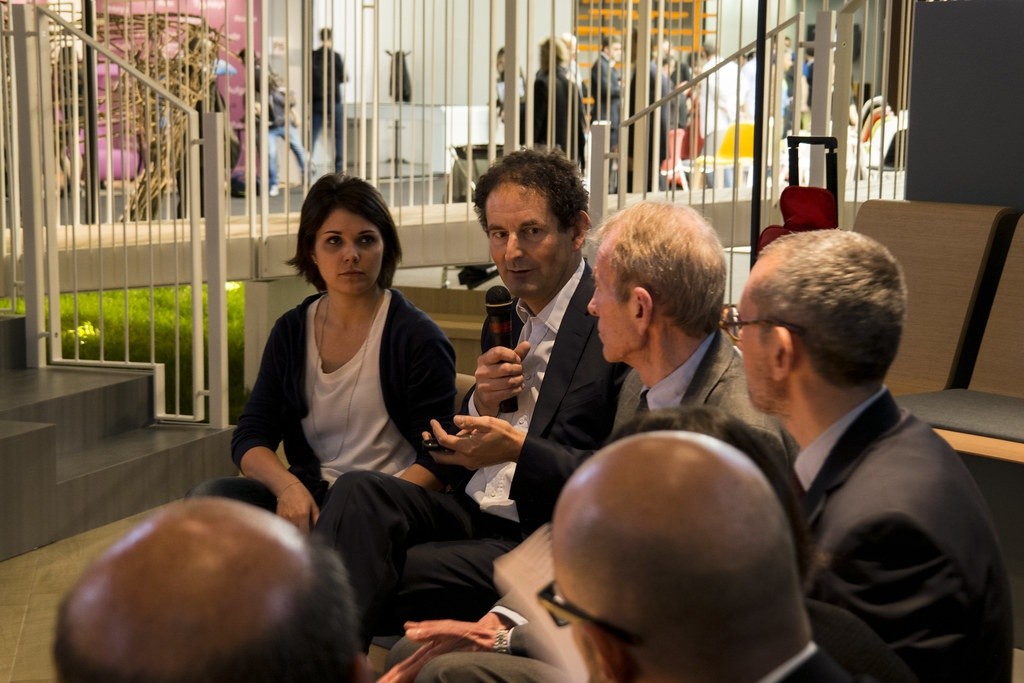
[313,150,633,661]
[553,430,855,683]
[613,407,913,683]
[735,229,1014,683]
[769,48,858,140]
[313,28,350,176]
[378,201,801,683]
[54,498,374,683]
[496,47,525,146]
[197,173,457,535]
[239,48,316,196]
[533,34,588,174]
[590,29,758,195]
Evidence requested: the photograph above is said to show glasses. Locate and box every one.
[539,579,646,647]
[720,303,809,342]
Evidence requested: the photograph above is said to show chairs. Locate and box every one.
[660,95,897,190]
[853,200,1024,462]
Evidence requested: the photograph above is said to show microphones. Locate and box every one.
[485,286,519,415]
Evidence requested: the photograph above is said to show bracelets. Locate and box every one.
[494,630,508,653]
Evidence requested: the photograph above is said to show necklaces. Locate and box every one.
[310,290,381,462]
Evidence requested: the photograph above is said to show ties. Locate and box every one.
[634,388,649,416]
